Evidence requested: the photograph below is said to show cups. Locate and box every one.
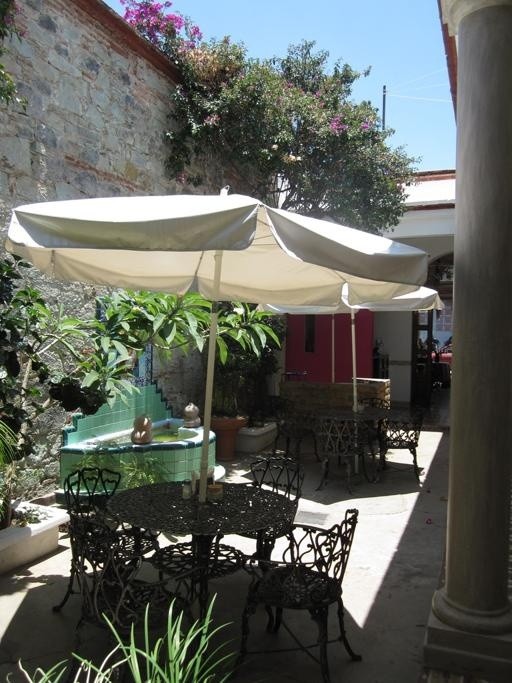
[206,484,223,503]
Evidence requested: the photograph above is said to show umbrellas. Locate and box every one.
[255,286,445,475]
[5,187,433,576]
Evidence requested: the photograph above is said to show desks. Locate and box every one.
[107,480,297,669]
[323,407,386,478]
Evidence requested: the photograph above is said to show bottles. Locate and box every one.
[183,480,191,500]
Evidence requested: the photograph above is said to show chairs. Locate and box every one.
[374,409,424,486]
[69,525,206,683]
[316,417,371,495]
[52,468,173,613]
[239,509,362,683]
[360,398,390,470]
[251,449,304,565]
[272,398,320,464]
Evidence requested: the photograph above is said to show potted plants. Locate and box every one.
[202,301,287,461]
